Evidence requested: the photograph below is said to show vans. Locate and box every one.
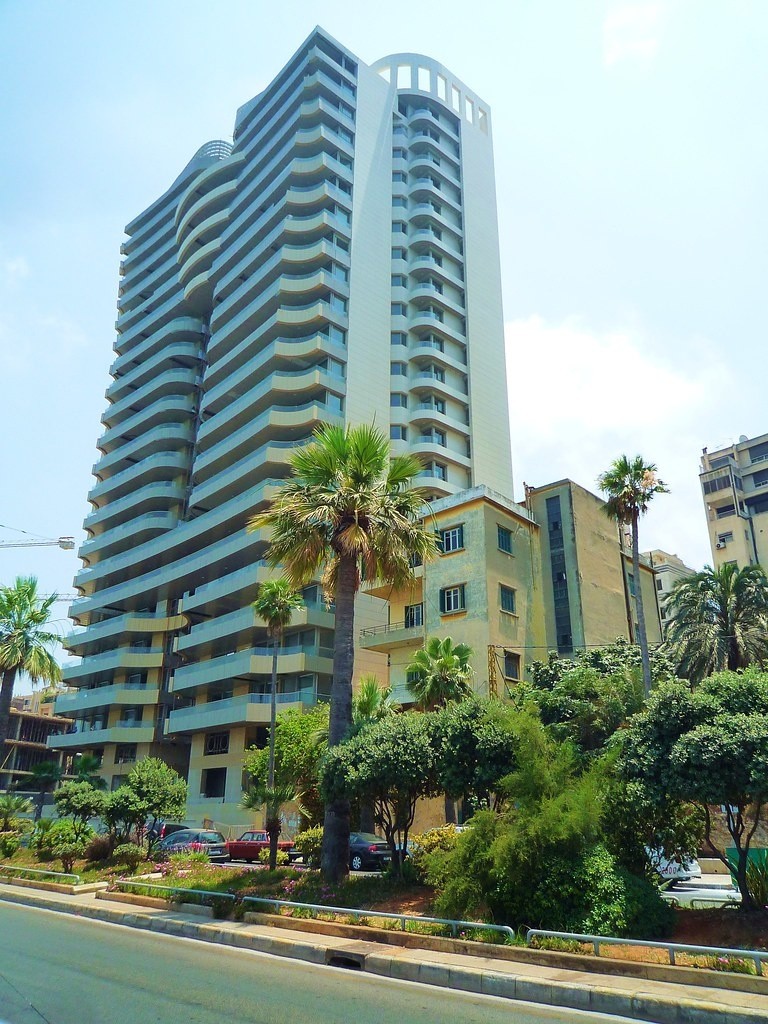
[145,821,190,846]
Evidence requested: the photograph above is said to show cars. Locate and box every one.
[150,827,230,867]
[643,842,702,883]
[304,832,392,871]
[395,841,422,860]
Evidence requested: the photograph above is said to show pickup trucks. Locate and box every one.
[228,829,296,865]
